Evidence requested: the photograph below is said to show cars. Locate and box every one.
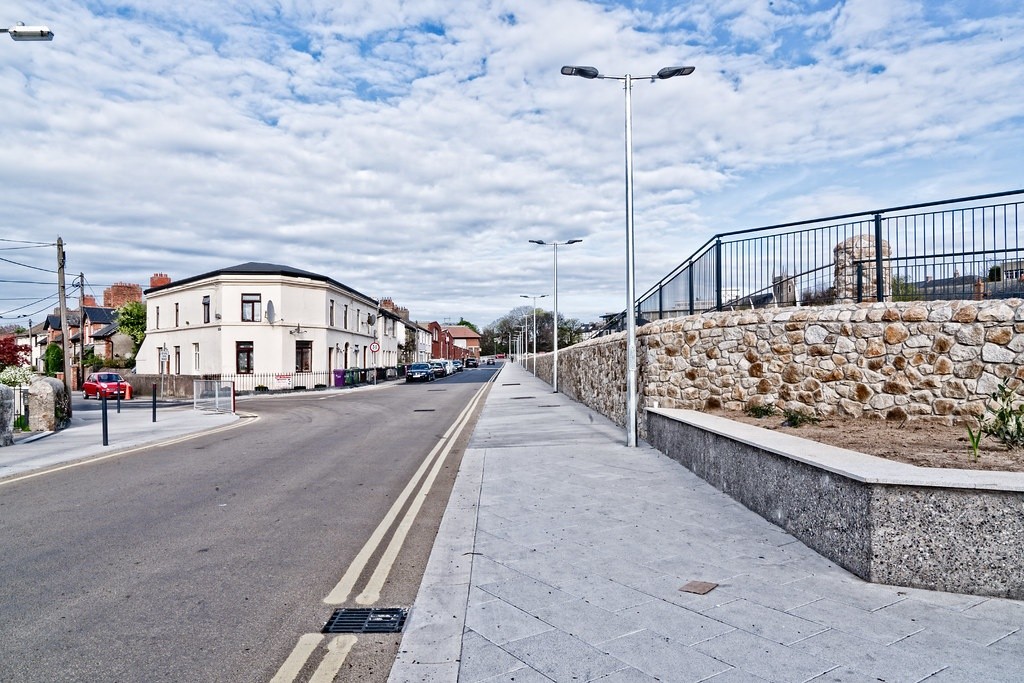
[81,372,133,400]
[487,359,495,365]
[466,358,478,368]
[405,358,463,383]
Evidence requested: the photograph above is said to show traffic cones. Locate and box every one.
[124,384,131,400]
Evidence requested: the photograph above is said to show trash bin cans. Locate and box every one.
[333,367,382,387]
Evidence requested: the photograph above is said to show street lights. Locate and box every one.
[519,295,546,379]
[510,314,534,371]
[101,339,113,361]
[561,65,697,448]
[528,239,583,394]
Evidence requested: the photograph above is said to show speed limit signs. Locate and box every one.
[370,342,380,352]
